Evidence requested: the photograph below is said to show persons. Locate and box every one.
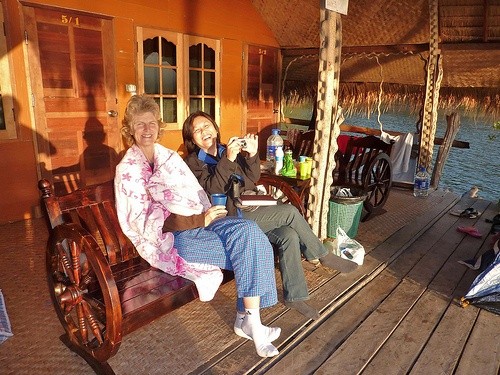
[114,95,281,358]
[182,110,358,319]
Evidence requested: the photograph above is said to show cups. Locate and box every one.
[284,150,293,172]
[305,158,313,179]
[299,162,306,180]
[210,194,227,206]
[299,156,307,162]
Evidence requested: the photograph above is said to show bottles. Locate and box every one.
[274,147,284,176]
[413,168,430,197]
[266,129,284,168]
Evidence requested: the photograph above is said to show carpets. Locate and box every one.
[0,176,445,375]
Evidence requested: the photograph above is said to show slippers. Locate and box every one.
[458,208,481,216]
[450,210,477,218]
[457,225,483,237]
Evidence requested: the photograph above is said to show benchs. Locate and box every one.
[293,129,395,214]
[38,176,306,375]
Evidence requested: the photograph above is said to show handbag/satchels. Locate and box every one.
[332,226,365,266]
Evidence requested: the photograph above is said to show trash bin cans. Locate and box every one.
[327,185,368,239]
